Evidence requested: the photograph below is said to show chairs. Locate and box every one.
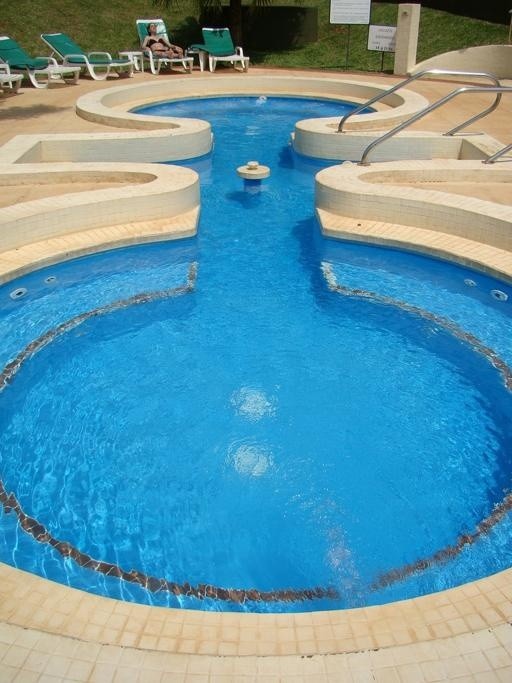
[136,18,250,74]
[0,32,136,98]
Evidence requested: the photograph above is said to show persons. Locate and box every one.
[141,22,184,58]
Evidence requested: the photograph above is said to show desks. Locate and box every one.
[118,50,144,73]
